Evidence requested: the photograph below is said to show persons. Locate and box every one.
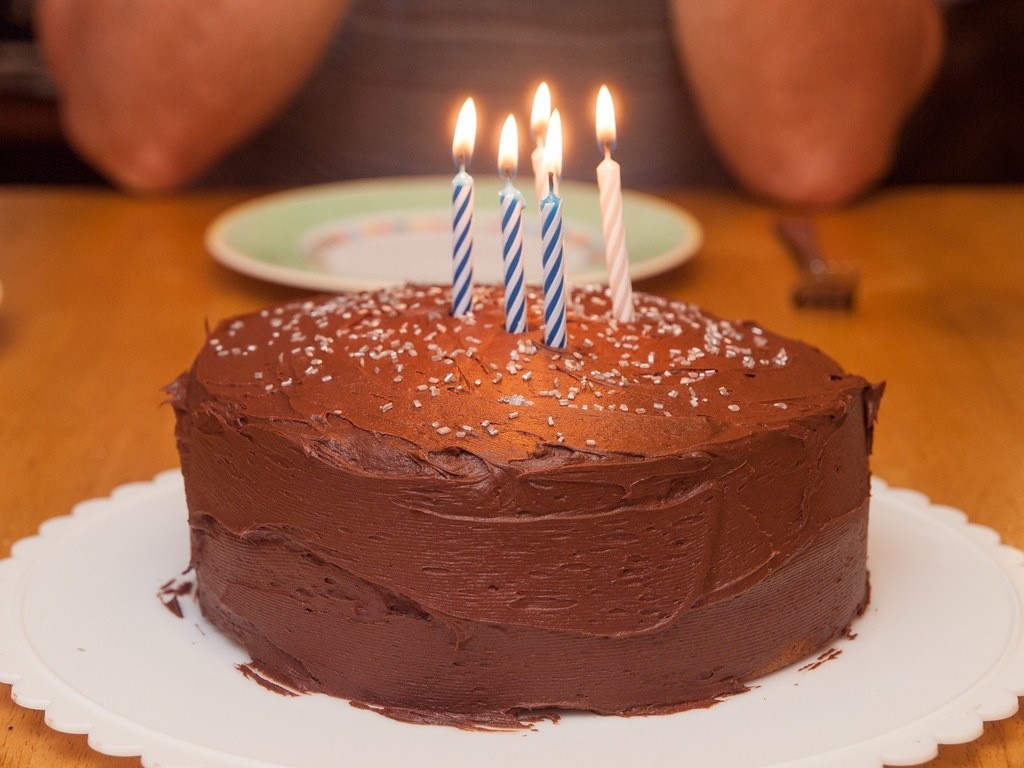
[32,0,946,210]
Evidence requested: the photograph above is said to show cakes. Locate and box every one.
[169,279,887,715]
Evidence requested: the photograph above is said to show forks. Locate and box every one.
[771,210,858,311]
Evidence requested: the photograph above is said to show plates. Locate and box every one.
[0,462,1024,768]
[207,174,704,294]
